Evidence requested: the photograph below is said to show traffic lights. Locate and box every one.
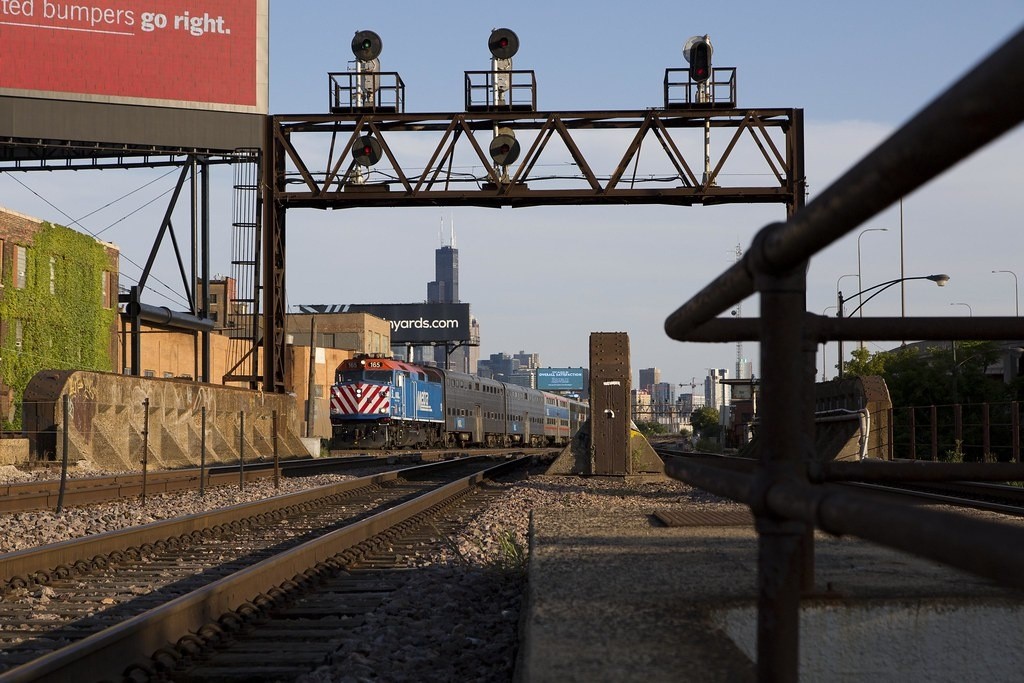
[351,135,384,167]
[689,41,712,82]
[488,134,521,166]
[351,30,383,62]
[487,28,520,59]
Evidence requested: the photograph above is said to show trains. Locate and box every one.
[325,352,590,452]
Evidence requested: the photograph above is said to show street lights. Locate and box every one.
[857,227,889,351]
[991,270,1019,318]
[950,302,972,317]
[832,274,952,378]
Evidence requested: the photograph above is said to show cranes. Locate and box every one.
[678,376,705,396]
